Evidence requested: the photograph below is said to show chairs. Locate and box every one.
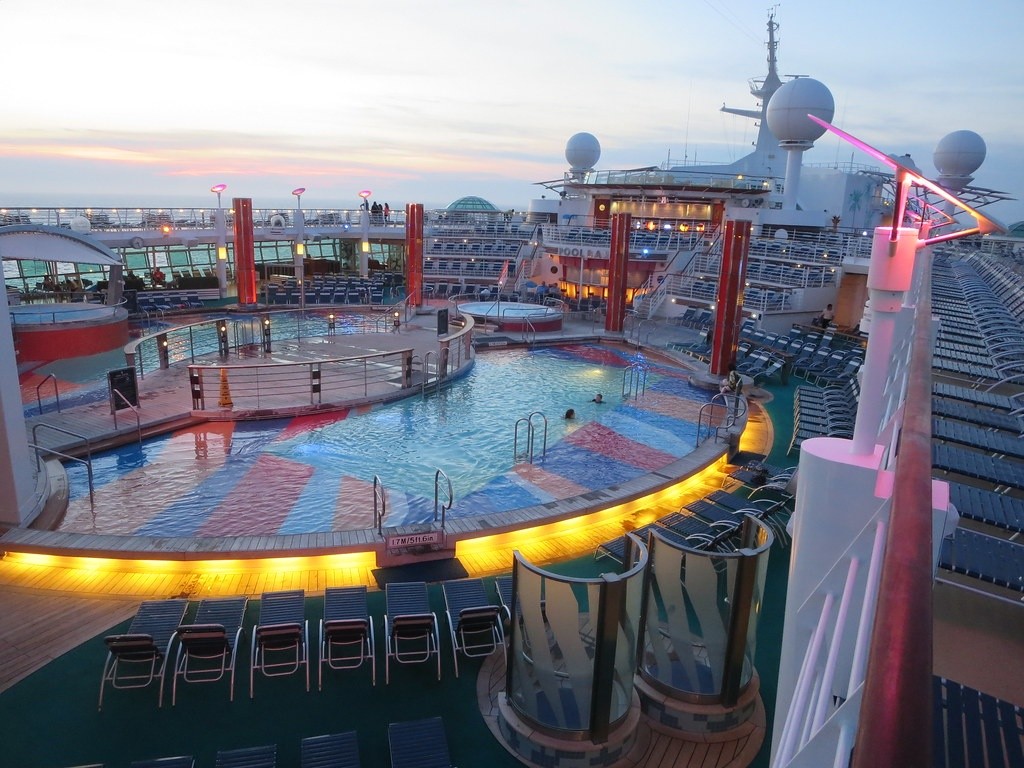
[410,213,533,304]
[71,716,454,768]
[3,211,395,228]
[440,578,508,677]
[96,600,189,712]
[267,273,387,308]
[172,596,254,706]
[493,574,549,631]
[924,244,1024,768]
[249,589,310,700]
[316,585,377,693]
[562,225,893,579]
[380,582,441,686]
[73,289,208,321]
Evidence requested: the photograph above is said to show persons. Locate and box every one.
[714,364,739,399]
[592,394,603,403]
[384,203,389,226]
[66,280,78,291]
[153,268,164,284]
[44,276,50,284]
[360,200,369,211]
[371,202,384,226]
[818,304,833,334]
[565,409,576,419]
[504,209,514,221]
[128,271,136,279]
